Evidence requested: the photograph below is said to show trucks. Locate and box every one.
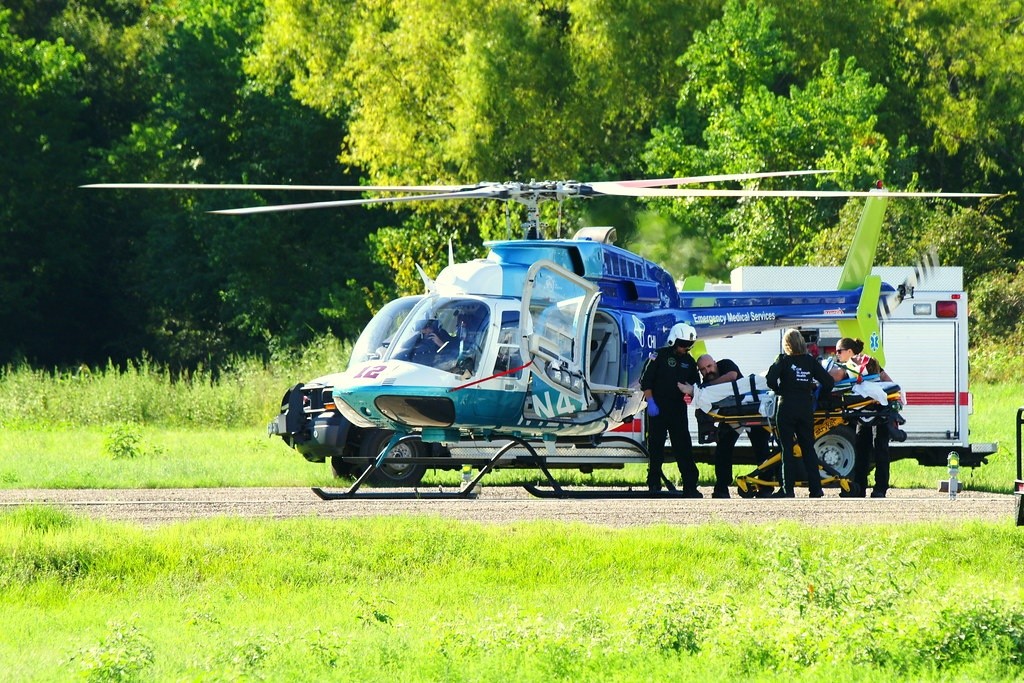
[272,262,978,483]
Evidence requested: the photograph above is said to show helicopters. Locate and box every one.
[77,166,1003,503]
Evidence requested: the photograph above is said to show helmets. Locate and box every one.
[412,310,442,333]
[667,323,697,351]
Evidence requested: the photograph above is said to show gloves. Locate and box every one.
[647,396,659,416]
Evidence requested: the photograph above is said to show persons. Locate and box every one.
[407,316,456,367]
[677,354,775,497]
[826,337,901,497]
[638,324,703,498]
[764,328,836,498]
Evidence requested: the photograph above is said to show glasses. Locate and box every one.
[835,348,847,354]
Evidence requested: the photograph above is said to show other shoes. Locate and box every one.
[773,491,795,498]
[712,485,730,498]
[648,485,660,491]
[683,488,703,498]
[840,491,866,497]
[756,489,775,498]
[809,488,823,496]
[871,489,885,497]
